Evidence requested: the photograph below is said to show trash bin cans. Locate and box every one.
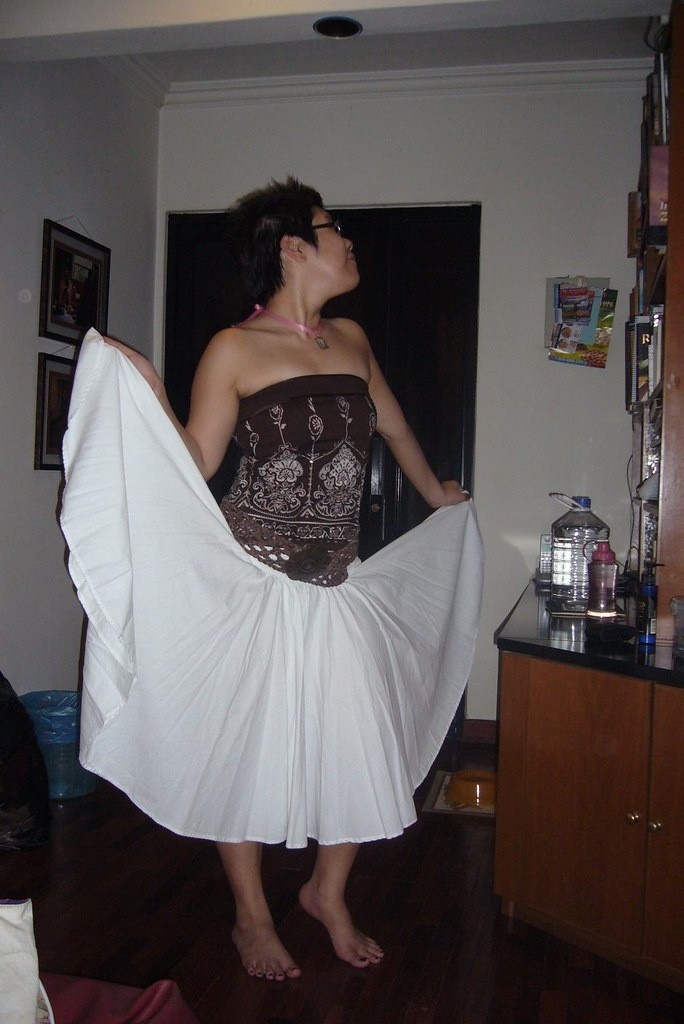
[20,690,98,799]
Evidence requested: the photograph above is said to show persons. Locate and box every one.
[59,176,485,981]
[63,268,95,325]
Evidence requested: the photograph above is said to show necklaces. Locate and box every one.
[234,304,329,350]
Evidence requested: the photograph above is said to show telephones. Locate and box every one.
[535,533,552,585]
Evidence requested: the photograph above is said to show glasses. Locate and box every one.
[293,220,342,235]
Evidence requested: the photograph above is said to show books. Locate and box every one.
[625,52,669,413]
[545,600,626,617]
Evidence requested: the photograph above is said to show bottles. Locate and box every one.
[635,561,664,645]
[547,492,610,612]
[581,540,617,612]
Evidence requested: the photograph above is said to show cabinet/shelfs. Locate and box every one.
[493,0,684,998]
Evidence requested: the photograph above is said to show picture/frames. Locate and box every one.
[39,218,111,346]
[33,353,75,470]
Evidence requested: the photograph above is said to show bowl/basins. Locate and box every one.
[446,768,494,808]
